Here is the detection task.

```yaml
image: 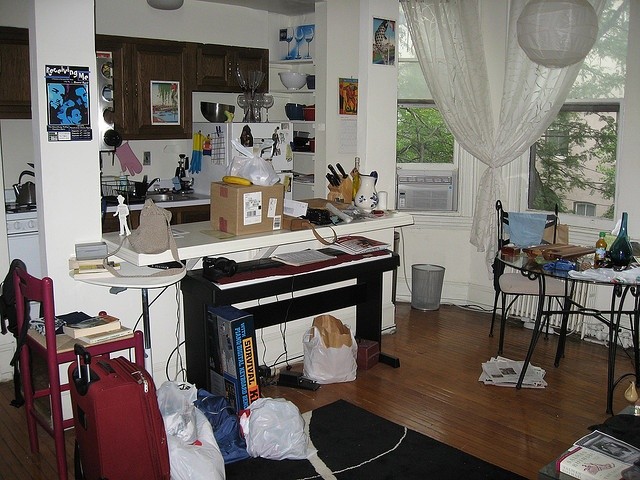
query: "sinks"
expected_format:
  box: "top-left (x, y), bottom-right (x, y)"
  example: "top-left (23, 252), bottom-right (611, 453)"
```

top-left (147, 194), bottom-right (190, 203)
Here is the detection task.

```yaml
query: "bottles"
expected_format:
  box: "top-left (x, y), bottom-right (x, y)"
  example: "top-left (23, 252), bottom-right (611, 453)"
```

top-left (594, 231), bottom-right (607, 269)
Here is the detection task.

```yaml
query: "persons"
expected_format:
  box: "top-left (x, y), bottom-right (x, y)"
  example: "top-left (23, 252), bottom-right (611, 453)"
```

top-left (48, 82), bottom-right (88, 124)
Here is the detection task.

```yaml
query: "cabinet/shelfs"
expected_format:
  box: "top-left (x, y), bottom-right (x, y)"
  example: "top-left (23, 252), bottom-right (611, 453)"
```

top-left (95, 33), bottom-right (187, 139)
top-left (0, 25), bottom-right (31, 119)
top-left (190, 41), bottom-right (269, 92)
top-left (268, 12), bottom-right (315, 202)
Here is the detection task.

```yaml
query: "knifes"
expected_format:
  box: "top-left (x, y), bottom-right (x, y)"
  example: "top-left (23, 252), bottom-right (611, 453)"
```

top-left (324, 162), bottom-right (353, 203)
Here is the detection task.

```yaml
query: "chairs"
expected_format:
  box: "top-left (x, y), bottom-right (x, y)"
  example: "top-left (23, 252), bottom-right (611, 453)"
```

top-left (12, 263), bottom-right (146, 480)
top-left (488, 200), bottom-right (574, 368)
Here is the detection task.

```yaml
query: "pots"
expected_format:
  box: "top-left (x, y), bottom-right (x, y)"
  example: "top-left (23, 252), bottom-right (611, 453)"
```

top-left (302, 104), bottom-right (315, 121)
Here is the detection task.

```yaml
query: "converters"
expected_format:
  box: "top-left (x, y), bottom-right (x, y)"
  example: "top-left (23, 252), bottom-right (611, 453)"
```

top-left (278, 370), bottom-right (303, 389)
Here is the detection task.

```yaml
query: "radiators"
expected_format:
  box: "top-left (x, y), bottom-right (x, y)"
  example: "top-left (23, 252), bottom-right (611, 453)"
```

top-left (503, 242), bottom-right (596, 339)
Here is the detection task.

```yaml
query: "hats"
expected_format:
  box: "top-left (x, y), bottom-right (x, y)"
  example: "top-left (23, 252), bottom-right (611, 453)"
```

top-left (300, 209), bottom-right (332, 224)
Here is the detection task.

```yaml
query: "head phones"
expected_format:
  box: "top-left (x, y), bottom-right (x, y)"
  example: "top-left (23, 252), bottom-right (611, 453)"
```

top-left (202, 256), bottom-right (238, 279)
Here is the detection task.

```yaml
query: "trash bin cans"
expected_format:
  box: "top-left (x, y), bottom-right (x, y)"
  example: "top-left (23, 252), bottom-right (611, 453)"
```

top-left (410, 263), bottom-right (446, 312)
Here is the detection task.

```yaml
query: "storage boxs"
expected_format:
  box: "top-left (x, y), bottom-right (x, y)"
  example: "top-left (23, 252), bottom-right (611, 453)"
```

top-left (210, 181), bottom-right (285, 236)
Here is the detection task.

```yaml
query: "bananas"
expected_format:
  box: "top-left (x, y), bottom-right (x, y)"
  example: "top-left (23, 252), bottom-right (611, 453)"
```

top-left (222, 176), bottom-right (253, 186)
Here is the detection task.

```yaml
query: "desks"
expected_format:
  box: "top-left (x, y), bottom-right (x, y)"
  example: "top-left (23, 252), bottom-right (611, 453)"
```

top-left (68, 260), bottom-right (188, 380)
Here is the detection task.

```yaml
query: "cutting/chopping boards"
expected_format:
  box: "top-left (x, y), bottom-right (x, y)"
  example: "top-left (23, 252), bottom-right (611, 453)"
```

top-left (145, 194), bottom-right (177, 202)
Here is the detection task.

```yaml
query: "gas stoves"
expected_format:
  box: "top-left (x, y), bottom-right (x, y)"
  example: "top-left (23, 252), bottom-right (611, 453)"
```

top-left (3, 188), bottom-right (42, 235)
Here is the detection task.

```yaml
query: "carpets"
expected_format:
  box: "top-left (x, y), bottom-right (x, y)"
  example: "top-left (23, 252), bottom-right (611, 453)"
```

top-left (225, 399), bottom-right (529, 480)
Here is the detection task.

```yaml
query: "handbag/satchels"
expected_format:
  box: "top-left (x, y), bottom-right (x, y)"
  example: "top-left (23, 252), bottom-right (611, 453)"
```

top-left (195, 389), bottom-right (250, 465)
top-left (309, 315), bottom-right (353, 348)
top-left (523, 243), bottom-right (596, 262)
top-left (126, 198), bottom-right (172, 254)
top-left (29, 316), bottom-right (67, 337)
top-left (281, 212), bottom-right (316, 231)
top-left (542, 214), bottom-right (568, 245)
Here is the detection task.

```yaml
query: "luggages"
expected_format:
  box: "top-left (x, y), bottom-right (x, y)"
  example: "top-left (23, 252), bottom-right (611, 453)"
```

top-left (68, 343), bottom-right (171, 480)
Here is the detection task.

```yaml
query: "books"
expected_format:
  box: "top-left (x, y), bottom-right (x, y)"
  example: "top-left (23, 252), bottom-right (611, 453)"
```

top-left (330, 231), bottom-right (391, 255)
top-left (72, 270), bottom-right (114, 279)
top-left (271, 248), bottom-right (338, 267)
top-left (574, 428), bottom-right (640, 466)
top-left (555, 445), bottom-right (633, 479)
top-left (78, 327), bottom-right (132, 345)
top-left (78, 262), bottom-right (121, 272)
top-left (62, 312), bottom-right (121, 339)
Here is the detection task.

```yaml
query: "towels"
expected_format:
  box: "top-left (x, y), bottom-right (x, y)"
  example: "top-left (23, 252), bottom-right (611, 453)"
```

top-left (503, 211), bottom-right (547, 248)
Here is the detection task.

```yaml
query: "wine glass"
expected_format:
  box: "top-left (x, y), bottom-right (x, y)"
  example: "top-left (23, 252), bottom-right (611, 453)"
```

top-left (253, 95), bottom-right (262, 123)
top-left (237, 95), bottom-right (248, 122)
top-left (236, 71), bottom-right (265, 122)
top-left (304, 28), bottom-right (313, 59)
top-left (263, 95), bottom-right (274, 122)
top-left (294, 27), bottom-right (303, 59)
top-left (286, 27), bottom-right (293, 60)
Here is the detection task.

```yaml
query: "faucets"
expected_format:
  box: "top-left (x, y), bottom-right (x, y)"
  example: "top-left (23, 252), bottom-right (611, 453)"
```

top-left (146, 178), bottom-right (160, 190)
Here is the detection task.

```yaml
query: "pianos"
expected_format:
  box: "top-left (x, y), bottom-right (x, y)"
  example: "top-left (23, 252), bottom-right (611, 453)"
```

top-left (181, 248), bottom-right (400, 391)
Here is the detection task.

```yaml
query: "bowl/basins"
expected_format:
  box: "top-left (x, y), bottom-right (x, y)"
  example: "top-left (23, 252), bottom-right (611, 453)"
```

top-left (284, 103), bottom-right (306, 120)
top-left (158, 188), bottom-right (170, 194)
top-left (200, 101), bottom-right (235, 123)
top-left (277, 72), bottom-right (307, 90)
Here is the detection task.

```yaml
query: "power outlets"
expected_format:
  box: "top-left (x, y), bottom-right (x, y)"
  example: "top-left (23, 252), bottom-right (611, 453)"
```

top-left (143, 151), bottom-right (150, 165)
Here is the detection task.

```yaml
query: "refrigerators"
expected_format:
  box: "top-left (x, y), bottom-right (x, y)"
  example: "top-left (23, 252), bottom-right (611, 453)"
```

top-left (192, 122), bottom-right (293, 199)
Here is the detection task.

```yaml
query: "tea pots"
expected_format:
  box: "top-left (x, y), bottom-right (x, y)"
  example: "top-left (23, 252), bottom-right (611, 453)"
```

top-left (13, 170), bottom-right (36, 205)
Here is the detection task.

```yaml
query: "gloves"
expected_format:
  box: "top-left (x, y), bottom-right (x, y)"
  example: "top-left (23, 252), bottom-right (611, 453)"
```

top-left (188, 133), bottom-right (206, 174)
top-left (115, 141), bottom-right (142, 176)
